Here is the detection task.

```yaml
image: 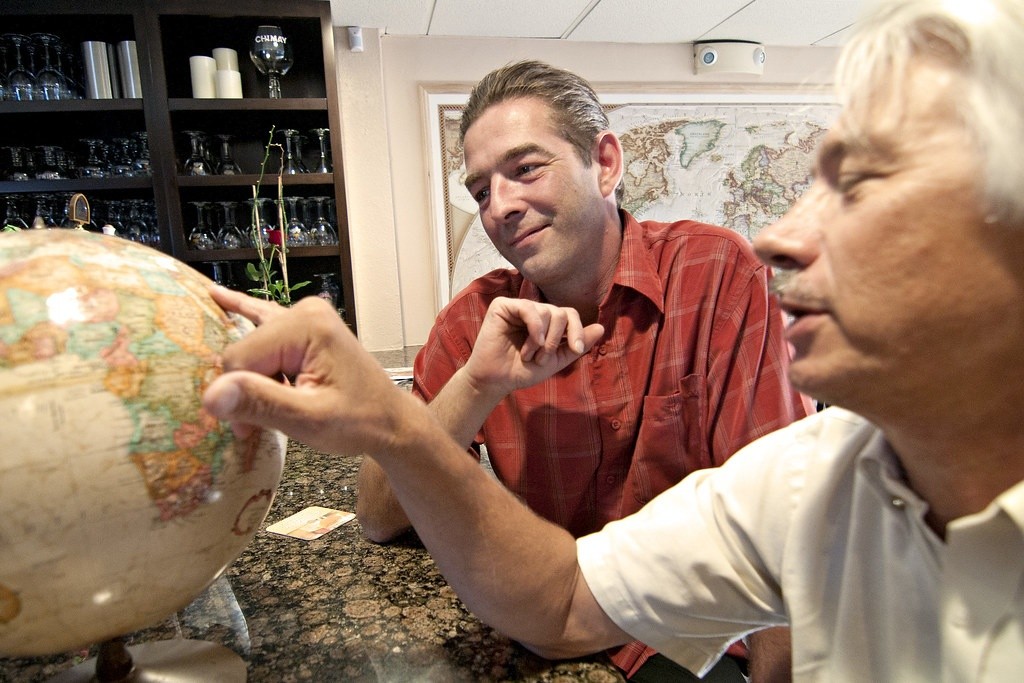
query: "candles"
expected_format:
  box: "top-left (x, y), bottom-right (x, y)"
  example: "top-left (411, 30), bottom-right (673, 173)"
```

top-left (212, 49), bottom-right (240, 72)
top-left (189, 56), bottom-right (217, 99)
top-left (215, 69), bottom-right (244, 99)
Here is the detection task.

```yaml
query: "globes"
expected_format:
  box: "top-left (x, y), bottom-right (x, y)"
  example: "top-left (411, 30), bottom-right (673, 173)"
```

top-left (0, 227), bottom-right (292, 683)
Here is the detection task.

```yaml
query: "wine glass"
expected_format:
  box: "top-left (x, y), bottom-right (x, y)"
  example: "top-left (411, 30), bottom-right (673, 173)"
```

top-left (0, 132), bottom-right (151, 179)
top-left (203, 262), bottom-right (355, 332)
top-left (182, 128), bottom-right (242, 177)
top-left (2, 194), bottom-right (157, 246)
top-left (190, 196), bottom-right (337, 247)
top-left (0, 33), bottom-right (85, 103)
top-left (276, 126), bottom-right (332, 174)
top-left (248, 24), bottom-right (291, 98)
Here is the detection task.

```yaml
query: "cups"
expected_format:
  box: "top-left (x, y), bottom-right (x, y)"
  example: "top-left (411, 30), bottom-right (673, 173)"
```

top-left (189, 47), bottom-right (244, 99)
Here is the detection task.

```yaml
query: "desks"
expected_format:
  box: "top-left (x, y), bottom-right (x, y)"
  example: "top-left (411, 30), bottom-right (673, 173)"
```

top-left (0, 438), bottom-right (633, 683)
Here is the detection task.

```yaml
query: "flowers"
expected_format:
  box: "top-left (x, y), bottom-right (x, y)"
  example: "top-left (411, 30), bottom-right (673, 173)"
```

top-left (244, 126), bottom-right (312, 306)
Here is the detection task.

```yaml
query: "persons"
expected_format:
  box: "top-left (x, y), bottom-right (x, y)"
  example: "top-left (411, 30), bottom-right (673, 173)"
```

top-left (204, 0), bottom-right (1024, 683)
top-left (357, 60), bottom-right (818, 683)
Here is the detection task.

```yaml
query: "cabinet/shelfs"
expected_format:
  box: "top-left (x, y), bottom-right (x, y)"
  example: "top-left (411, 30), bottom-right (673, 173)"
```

top-left (0, 0), bottom-right (358, 339)
